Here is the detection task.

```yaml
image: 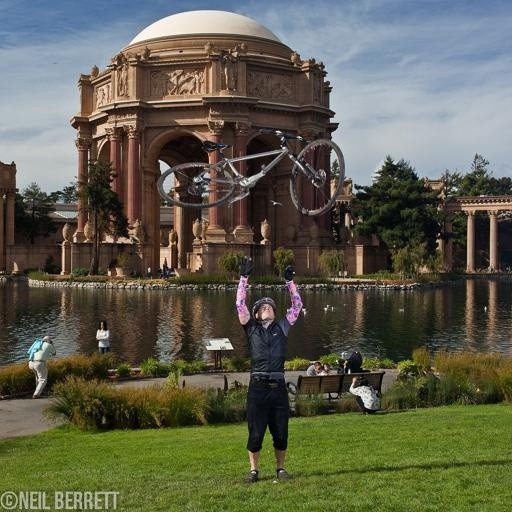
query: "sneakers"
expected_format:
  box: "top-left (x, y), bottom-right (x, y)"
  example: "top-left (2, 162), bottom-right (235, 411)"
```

top-left (249, 470), bottom-right (257, 482)
top-left (277, 468), bottom-right (290, 481)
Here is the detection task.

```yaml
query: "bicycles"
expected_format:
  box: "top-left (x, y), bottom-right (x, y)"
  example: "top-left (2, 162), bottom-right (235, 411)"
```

top-left (155, 124), bottom-right (346, 218)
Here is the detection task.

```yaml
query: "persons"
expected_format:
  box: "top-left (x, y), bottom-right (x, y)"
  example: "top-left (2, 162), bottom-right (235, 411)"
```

top-left (96, 320), bottom-right (111, 353)
top-left (235, 255), bottom-right (303, 482)
top-left (28, 336), bottom-right (56, 398)
top-left (349, 376), bottom-right (381, 415)
top-left (147, 265), bottom-right (170, 279)
top-left (307, 353), bottom-right (371, 378)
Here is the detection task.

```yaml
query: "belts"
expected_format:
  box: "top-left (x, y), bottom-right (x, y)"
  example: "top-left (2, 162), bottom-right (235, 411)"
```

top-left (254, 382), bottom-right (278, 388)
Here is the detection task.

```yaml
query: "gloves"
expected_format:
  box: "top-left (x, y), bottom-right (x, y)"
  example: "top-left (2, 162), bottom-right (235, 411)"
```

top-left (238, 255), bottom-right (255, 276)
top-left (284, 265), bottom-right (296, 280)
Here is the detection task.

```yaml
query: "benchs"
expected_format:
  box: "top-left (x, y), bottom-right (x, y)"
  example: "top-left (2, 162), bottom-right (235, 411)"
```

top-left (338, 370), bottom-right (387, 400)
top-left (285, 372), bottom-right (345, 406)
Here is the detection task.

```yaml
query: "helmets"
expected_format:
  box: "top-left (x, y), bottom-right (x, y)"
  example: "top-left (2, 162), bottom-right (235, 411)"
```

top-left (252, 297), bottom-right (277, 320)
